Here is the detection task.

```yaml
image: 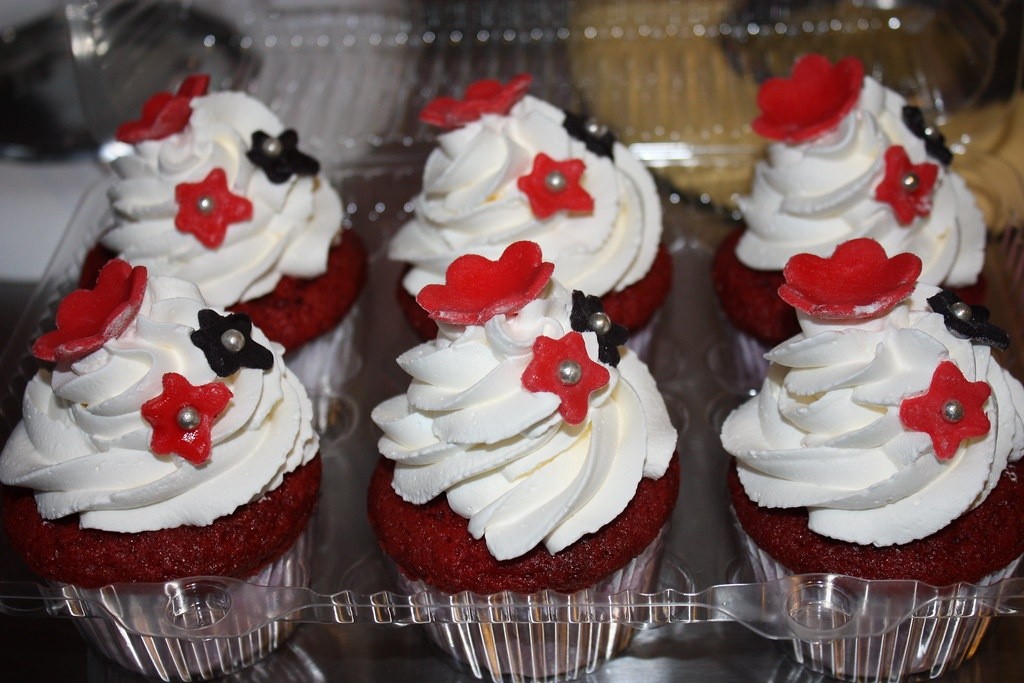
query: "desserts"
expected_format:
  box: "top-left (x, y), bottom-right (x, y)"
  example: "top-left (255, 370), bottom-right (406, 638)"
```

top-left (0, 53), bottom-right (1024, 677)
